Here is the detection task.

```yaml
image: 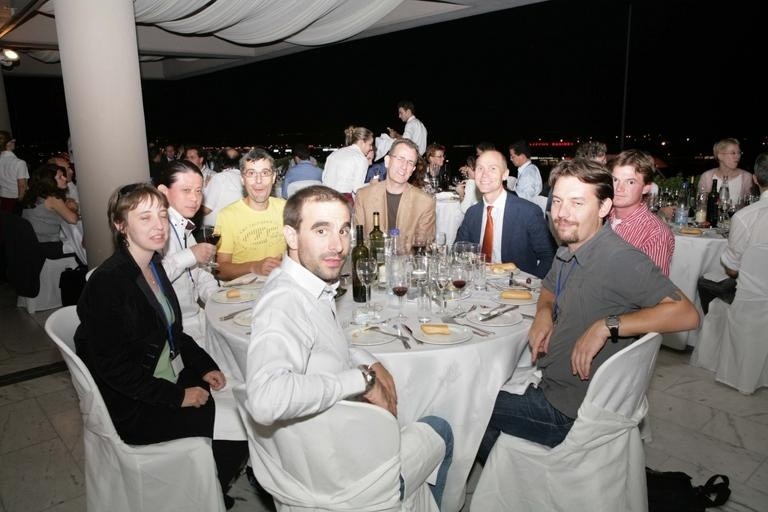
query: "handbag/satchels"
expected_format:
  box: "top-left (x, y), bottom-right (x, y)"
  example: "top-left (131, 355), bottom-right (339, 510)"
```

top-left (643, 464), bottom-right (732, 512)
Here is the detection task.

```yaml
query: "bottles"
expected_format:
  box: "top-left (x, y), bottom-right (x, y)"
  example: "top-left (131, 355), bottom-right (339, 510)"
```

top-left (424, 161), bottom-right (469, 200)
top-left (644, 175), bottom-right (755, 234)
top-left (351, 211), bottom-right (387, 303)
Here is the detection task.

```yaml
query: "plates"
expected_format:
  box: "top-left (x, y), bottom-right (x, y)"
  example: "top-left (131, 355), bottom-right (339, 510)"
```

top-left (495, 278), bottom-right (540, 290)
top-left (233, 308), bottom-right (253, 326)
top-left (493, 290), bottom-right (538, 306)
top-left (413, 323), bottom-right (473, 344)
top-left (213, 289), bottom-right (257, 303)
top-left (490, 263), bottom-right (520, 278)
top-left (345, 324), bottom-right (399, 345)
top-left (466, 308), bottom-right (522, 325)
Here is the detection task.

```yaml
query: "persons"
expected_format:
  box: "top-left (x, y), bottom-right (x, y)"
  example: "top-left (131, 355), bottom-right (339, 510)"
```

top-left (388, 100), bottom-right (429, 156)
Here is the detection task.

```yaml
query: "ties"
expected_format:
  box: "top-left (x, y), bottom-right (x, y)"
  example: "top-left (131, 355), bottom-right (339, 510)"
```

top-left (478, 205), bottom-right (498, 265)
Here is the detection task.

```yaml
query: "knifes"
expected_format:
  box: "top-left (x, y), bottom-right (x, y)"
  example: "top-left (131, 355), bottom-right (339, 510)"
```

top-left (401, 323), bottom-right (424, 345)
top-left (480, 305), bottom-right (519, 322)
top-left (509, 272), bottom-right (514, 285)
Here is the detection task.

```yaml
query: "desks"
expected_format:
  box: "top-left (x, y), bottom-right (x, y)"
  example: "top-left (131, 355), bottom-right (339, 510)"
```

top-left (60, 216), bottom-right (88, 265)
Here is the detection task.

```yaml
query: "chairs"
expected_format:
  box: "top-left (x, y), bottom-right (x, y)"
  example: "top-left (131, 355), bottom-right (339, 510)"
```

top-left (44, 304), bottom-right (227, 512)
top-left (286, 178), bottom-right (321, 201)
top-left (84, 261), bottom-right (103, 282)
top-left (688, 214), bottom-right (768, 394)
top-left (15, 216), bottom-right (77, 314)
top-left (230, 382), bottom-right (442, 511)
top-left (533, 195), bottom-right (549, 218)
top-left (469, 328), bottom-right (670, 511)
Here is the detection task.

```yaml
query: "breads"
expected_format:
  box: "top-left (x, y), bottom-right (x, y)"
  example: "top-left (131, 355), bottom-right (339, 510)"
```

top-left (680, 228), bottom-right (703, 234)
top-left (421, 324), bottom-right (450, 335)
top-left (227, 288), bottom-right (239, 298)
top-left (491, 262), bottom-right (515, 270)
top-left (502, 291), bottom-right (532, 299)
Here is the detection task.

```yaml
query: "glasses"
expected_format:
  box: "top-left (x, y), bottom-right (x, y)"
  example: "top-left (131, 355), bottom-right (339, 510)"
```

top-left (386, 152), bottom-right (416, 169)
top-left (241, 169), bottom-right (275, 179)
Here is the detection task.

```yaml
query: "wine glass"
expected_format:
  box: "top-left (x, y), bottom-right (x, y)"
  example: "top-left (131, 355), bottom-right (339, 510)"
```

top-left (203, 226), bottom-right (222, 268)
top-left (356, 228), bottom-right (489, 322)
